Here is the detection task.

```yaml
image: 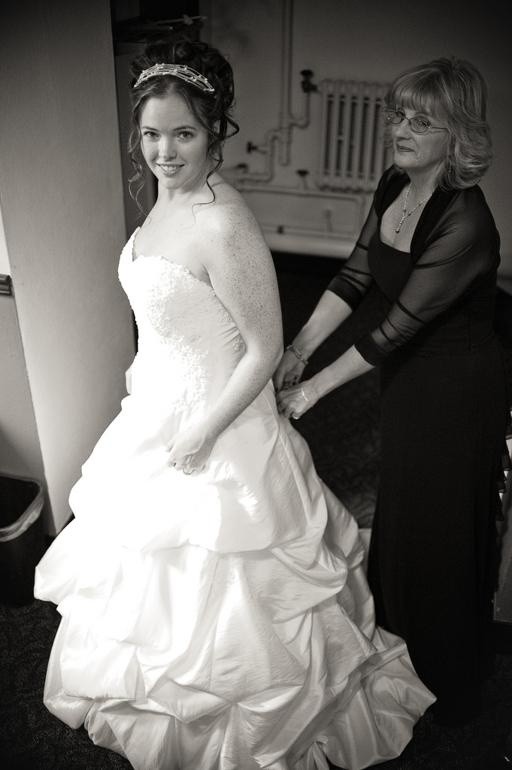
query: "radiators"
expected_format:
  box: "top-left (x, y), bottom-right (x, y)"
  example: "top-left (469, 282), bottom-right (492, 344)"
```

top-left (315, 79), bottom-right (392, 191)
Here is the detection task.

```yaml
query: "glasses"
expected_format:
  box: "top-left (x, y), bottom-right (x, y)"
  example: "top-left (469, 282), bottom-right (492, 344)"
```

top-left (386, 107), bottom-right (451, 136)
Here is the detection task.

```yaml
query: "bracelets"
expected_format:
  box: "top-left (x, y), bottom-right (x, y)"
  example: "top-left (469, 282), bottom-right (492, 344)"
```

top-left (286, 345), bottom-right (308, 365)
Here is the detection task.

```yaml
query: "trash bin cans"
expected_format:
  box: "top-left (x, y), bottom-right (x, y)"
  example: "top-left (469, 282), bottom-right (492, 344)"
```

top-left (0, 473), bottom-right (47, 608)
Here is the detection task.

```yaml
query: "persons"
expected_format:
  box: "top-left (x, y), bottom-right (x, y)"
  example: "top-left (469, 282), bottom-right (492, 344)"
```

top-left (273, 55), bottom-right (512, 729)
top-left (34, 20), bottom-right (438, 769)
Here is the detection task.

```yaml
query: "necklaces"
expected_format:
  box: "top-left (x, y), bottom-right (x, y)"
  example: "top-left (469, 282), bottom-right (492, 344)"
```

top-left (396, 181), bottom-right (432, 233)
